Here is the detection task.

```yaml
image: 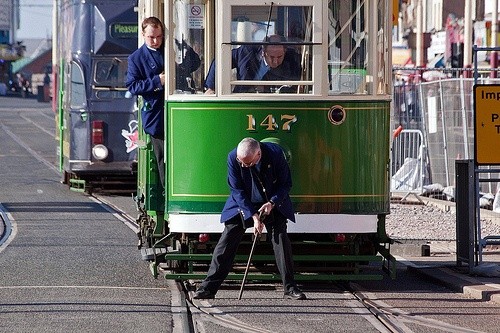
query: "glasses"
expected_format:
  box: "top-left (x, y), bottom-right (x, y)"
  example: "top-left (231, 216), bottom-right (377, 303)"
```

top-left (235, 155), bottom-right (257, 168)
top-left (148, 34), bottom-right (162, 39)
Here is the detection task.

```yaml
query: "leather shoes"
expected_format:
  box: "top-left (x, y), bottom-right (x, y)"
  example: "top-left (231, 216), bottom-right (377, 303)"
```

top-left (283, 288), bottom-right (306, 300)
top-left (193, 287), bottom-right (215, 299)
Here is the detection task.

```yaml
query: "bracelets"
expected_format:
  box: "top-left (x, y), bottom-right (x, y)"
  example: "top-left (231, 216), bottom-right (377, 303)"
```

top-left (269, 201), bottom-right (275, 206)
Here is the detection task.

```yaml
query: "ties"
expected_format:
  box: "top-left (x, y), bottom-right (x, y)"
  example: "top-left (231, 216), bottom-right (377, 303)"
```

top-left (250, 166), bottom-right (269, 201)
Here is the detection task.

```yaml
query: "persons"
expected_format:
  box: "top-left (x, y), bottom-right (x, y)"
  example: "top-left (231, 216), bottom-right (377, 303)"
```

top-left (125, 17), bottom-right (201, 189)
top-left (206, 34), bottom-right (302, 94)
top-left (193, 137), bottom-right (306, 300)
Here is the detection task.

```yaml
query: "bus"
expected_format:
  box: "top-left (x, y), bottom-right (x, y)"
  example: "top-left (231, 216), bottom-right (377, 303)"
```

top-left (52, 0), bottom-right (144, 190)
top-left (133, 0), bottom-right (393, 287)
